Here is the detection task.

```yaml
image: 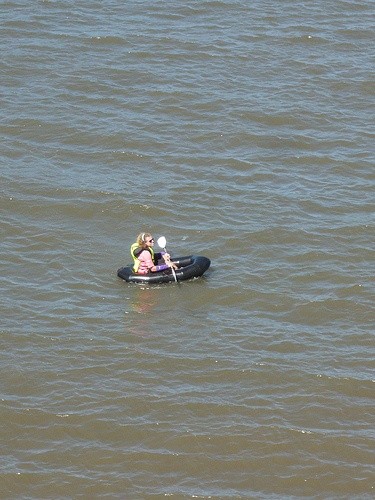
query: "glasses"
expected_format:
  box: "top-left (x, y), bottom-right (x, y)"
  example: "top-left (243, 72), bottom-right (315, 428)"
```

top-left (149, 238), bottom-right (154, 242)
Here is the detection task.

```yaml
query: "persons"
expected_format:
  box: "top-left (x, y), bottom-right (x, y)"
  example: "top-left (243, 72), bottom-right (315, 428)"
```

top-left (130, 232), bottom-right (178, 275)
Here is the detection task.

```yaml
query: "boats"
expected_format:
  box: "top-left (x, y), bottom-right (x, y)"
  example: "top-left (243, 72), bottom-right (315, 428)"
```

top-left (116, 255), bottom-right (211, 284)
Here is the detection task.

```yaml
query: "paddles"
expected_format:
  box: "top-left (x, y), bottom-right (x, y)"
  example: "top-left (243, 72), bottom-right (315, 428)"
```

top-left (157, 236), bottom-right (177, 283)
